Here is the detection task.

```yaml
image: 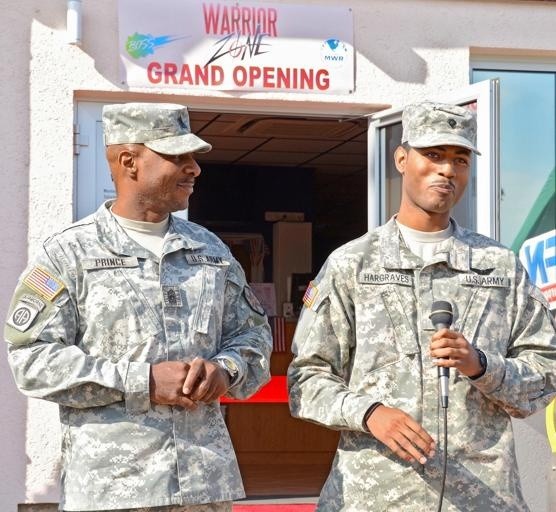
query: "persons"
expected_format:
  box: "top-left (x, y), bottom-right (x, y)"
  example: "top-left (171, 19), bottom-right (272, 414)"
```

top-left (285, 101), bottom-right (555, 510)
top-left (0, 101), bottom-right (276, 511)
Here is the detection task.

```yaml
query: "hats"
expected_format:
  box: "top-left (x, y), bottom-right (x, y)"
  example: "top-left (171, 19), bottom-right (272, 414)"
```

top-left (101, 103), bottom-right (213, 157)
top-left (402, 101), bottom-right (483, 157)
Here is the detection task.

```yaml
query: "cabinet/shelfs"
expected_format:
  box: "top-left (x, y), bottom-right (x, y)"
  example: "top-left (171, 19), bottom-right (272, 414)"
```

top-left (219, 349), bottom-right (342, 500)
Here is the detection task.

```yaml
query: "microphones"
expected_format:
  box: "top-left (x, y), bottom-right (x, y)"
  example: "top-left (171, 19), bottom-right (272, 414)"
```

top-left (429, 299), bottom-right (453, 412)
